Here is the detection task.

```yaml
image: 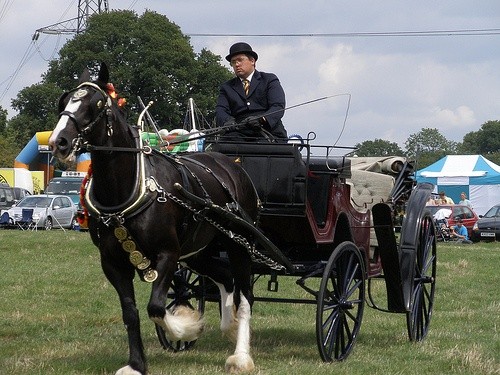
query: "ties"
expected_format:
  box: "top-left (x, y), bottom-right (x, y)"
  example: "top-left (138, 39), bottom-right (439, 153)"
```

top-left (244, 80), bottom-right (250, 96)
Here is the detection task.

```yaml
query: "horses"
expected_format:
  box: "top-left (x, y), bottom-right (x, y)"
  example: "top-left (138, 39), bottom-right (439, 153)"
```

top-left (45, 58), bottom-right (271, 375)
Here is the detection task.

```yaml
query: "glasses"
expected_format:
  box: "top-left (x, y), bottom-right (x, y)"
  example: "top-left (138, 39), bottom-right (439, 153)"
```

top-left (440, 194), bottom-right (444, 196)
top-left (231, 58), bottom-right (251, 65)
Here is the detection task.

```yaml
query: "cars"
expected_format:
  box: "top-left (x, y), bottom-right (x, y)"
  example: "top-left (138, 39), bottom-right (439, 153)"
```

top-left (425, 205), bottom-right (480, 243)
top-left (471, 204), bottom-right (500, 242)
top-left (5, 194), bottom-right (78, 231)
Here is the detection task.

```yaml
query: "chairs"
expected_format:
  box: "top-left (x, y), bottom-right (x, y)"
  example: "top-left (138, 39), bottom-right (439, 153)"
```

top-left (0, 208), bottom-right (38, 231)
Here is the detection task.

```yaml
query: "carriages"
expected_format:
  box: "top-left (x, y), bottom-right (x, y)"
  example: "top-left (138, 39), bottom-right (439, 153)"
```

top-left (47, 61), bottom-right (436, 375)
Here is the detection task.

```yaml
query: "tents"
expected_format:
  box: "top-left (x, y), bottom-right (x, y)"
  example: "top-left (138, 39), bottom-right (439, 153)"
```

top-left (408, 154), bottom-right (500, 219)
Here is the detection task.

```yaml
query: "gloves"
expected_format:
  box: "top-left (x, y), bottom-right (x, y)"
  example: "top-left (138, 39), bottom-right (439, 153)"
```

top-left (224, 120), bottom-right (239, 130)
top-left (242, 116), bottom-right (260, 127)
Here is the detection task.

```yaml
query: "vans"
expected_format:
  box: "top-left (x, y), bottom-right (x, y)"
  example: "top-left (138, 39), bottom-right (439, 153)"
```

top-left (0, 186), bottom-right (33, 217)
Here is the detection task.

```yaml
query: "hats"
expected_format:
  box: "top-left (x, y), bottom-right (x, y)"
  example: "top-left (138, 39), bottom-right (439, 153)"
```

top-left (225, 43), bottom-right (258, 62)
top-left (454, 217), bottom-right (460, 221)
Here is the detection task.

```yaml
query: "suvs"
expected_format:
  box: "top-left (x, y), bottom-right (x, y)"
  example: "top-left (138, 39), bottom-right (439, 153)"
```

top-left (42, 171), bottom-right (86, 215)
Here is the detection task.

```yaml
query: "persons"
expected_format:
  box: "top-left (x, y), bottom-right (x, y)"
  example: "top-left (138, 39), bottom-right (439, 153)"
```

top-left (440, 219), bottom-right (468, 241)
top-left (426, 192), bottom-right (473, 218)
top-left (216, 42), bottom-right (289, 144)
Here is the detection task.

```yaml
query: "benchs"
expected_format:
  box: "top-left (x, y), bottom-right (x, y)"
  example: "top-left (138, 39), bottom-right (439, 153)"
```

top-left (303, 158), bottom-right (351, 225)
top-left (346, 155), bottom-right (409, 249)
